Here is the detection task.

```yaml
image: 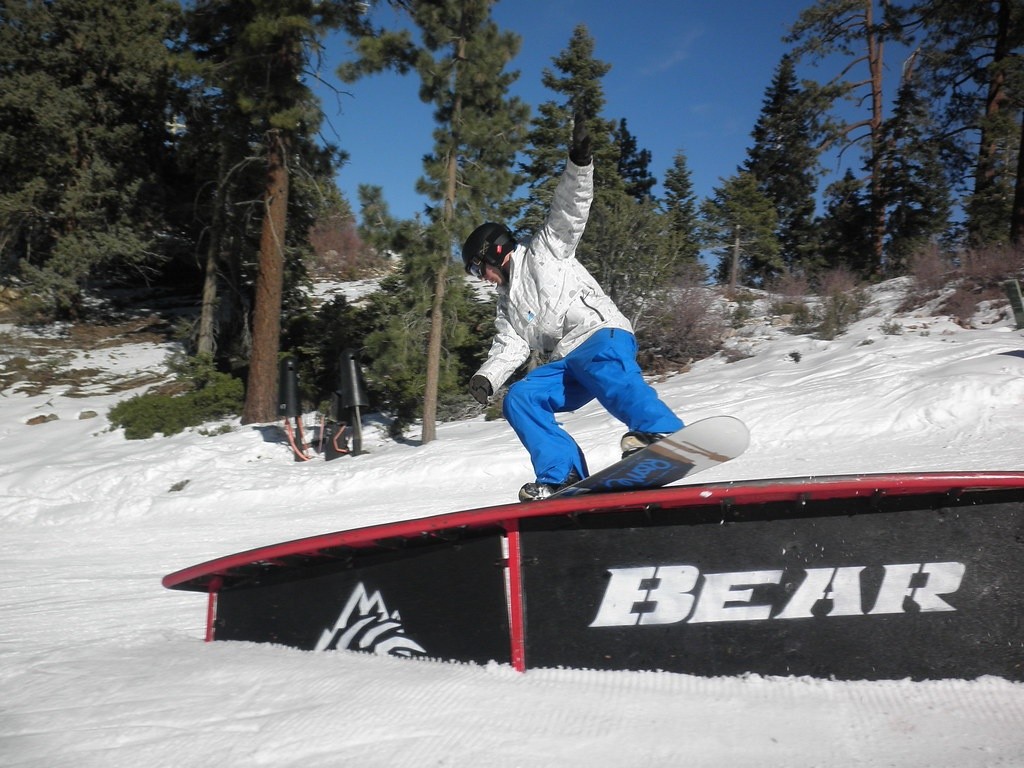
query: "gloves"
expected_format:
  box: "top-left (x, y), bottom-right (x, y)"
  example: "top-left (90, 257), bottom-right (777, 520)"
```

top-left (570, 112), bottom-right (592, 165)
top-left (468, 375), bottom-right (493, 405)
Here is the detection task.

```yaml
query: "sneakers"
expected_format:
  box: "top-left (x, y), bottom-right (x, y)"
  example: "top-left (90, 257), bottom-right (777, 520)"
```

top-left (518, 467), bottom-right (581, 502)
top-left (621, 430), bottom-right (672, 459)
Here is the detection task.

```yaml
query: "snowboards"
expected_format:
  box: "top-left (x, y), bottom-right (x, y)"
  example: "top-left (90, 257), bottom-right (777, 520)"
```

top-left (546, 415), bottom-right (751, 499)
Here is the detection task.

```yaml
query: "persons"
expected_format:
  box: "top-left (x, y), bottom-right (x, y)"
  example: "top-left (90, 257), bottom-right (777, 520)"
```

top-left (462, 111), bottom-right (684, 502)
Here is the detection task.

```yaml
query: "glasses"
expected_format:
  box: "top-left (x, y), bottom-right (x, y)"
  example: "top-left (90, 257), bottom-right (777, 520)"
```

top-left (465, 255), bottom-right (486, 280)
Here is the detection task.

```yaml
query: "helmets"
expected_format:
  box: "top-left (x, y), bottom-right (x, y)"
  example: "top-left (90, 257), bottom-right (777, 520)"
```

top-left (462, 222), bottom-right (518, 266)
